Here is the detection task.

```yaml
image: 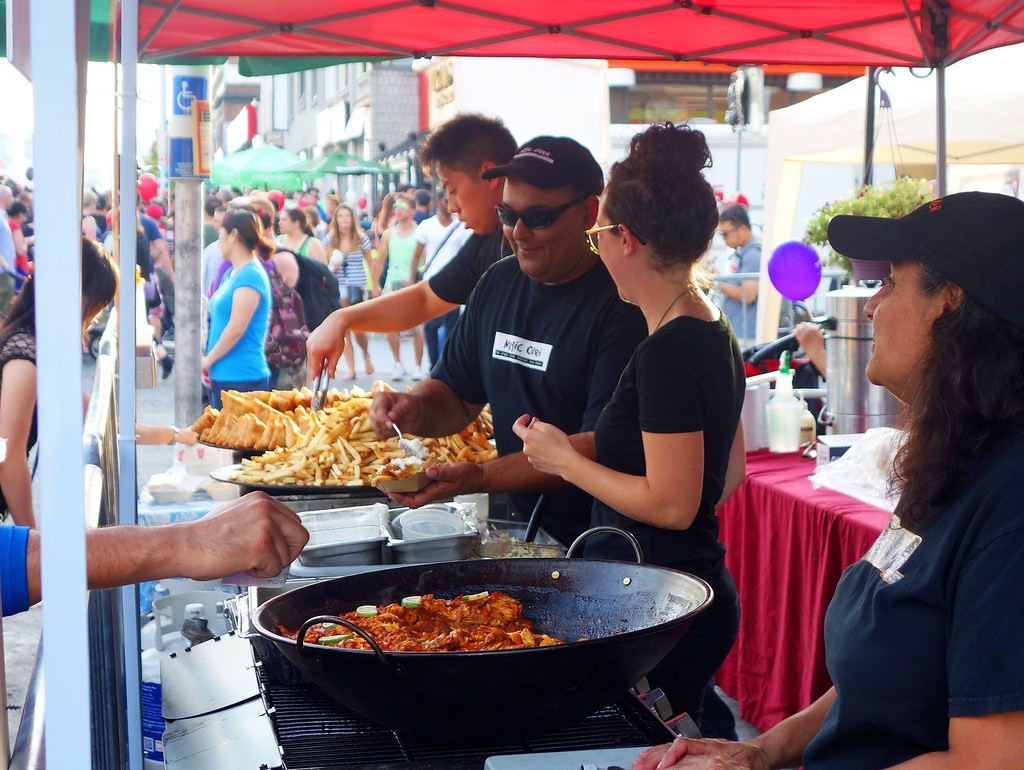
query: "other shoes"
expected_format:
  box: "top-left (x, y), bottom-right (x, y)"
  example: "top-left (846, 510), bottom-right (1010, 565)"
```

top-left (341, 370), bottom-right (356, 381)
top-left (391, 361), bottom-right (405, 381)
top-left (363, 352), bottom-right (373, 374)
top-left (412, 363), bottom-right (422, 380)
top-left (161, 353), bottom-right (174, 379)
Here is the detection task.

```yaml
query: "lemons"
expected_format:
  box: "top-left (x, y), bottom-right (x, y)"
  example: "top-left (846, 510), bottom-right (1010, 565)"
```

top-left (461, 591), bottom-right (489, 601)
top-left (401, 596), bottom-right (422, 608)
top-left (319, 635), bottom-right (353, 645)
top-left (356, 605), bottom-right (377, 616)
top-left (323, 623), bottom-right (337, 628)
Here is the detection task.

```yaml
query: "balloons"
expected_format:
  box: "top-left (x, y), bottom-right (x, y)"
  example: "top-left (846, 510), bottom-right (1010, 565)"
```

top-left (768, 242), bottom-right (823, 303)
top-left (357, 197), bottom-right (366, 210)
top-left (268, 191), bottom-right (285, 211)
top-left (137, 174), bottom-right (160, 201)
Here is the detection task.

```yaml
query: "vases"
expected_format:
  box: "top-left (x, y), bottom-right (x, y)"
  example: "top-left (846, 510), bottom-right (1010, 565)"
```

top-left (849, 258), bottom-right (890, 288)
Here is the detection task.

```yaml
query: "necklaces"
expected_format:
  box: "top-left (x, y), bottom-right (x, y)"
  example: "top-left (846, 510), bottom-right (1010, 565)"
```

top-left (652, 284), bottom-right (697, 333)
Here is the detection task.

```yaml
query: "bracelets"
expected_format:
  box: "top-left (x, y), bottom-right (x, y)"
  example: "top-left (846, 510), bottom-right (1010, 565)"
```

top-left (168, 425), bottom-right (181, 446)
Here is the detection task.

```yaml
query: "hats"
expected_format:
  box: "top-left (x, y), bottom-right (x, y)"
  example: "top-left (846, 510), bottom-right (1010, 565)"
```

top-left (827, 190), bottom-right (1024, 336)
top-left (480, 136), bottom-right (603, 196)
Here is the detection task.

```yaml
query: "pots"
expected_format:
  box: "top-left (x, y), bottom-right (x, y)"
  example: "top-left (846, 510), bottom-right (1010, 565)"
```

top-left (250, 526), bottom-right (715, 724)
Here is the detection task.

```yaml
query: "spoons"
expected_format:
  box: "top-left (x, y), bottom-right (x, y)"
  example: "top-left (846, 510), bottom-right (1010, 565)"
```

top-left (392, 422), bottom-right (413, 454)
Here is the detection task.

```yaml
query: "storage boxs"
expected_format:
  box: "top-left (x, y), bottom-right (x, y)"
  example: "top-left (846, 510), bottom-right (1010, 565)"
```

top-left (294, 502), bottom-right (388, 568)
top-left (815, 433), bottom-right (862, 466)
top-left (377, 503), bottom-right (476, 562)
top-left (471, 518), bottom-right (568, 557)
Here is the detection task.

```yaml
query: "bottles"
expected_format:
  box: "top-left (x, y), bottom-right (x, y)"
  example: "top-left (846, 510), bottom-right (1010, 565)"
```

top-left (799, 393), bottom-right (817, 445)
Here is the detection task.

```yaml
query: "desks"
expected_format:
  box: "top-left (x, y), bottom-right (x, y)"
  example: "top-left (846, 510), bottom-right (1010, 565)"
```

top-left (715, 449), bottom-right (893, 732)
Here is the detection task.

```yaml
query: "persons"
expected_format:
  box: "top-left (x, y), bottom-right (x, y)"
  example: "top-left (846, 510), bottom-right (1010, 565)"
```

top-left (0, 166), bottom-right (475, 412)
top-left (792, 321), bottom-right (827, 436)
top-left (695, 205), bottom-right (761, 339)
top-left (511, 121), bottom-right (743, 743)
top-left (0, 491), bottom-right (310, 617)
top-left (631, 191), bottom-right (1024, 770)
top-left (370, 136), bottom-right (647, 556)
top-left (0, 236), bottom-right (197, 530)
top-left (305, 113), bottom-right (519, 380)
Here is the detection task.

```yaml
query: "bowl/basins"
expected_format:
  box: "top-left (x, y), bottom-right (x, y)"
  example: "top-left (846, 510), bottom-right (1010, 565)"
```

top-left (200, 479), bottom-right (238, 500)
top-left (399, 509), bottom-right (465, 541)
top-left (145, 471), bottom-right (198, 503)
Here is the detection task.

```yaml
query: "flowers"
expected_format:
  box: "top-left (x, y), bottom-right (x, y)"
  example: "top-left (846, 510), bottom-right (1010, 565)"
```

top-left (800, 176), bottom-right (935, 243)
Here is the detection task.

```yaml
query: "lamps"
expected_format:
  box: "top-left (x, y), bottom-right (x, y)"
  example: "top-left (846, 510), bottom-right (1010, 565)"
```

top-left (608, 68), bottom-right (637, 90)
top-left (785, 72), bottom-right (823, 93)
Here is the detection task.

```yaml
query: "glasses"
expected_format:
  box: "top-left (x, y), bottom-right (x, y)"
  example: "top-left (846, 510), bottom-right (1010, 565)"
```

top-left (719, 222), bottom-right (744, 238)
top-left (584, 223), bottom-right (647, 255)
top-left (494, 191), bottom-right (592, 229)
top-left (392, 202), bottom-right (409, 211)
top-left (309, 192), bottom-right (319, 201)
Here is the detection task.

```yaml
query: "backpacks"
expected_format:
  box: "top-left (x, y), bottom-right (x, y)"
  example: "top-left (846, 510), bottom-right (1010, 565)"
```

top-left (259, 259), bottom-right (309, 369)
top-left (296, 250), bottom-right (341, 332)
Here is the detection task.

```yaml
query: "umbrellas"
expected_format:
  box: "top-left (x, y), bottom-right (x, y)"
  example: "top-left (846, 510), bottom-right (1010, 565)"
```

top-left (206, 143), bottom-right (400, 192)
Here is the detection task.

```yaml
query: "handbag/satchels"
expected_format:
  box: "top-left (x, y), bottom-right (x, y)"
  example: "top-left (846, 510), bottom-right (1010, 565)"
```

top-left (346, 285), bottom-right (363, 305)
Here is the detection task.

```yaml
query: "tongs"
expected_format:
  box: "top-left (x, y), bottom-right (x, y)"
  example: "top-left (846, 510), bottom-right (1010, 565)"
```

top-left (310, 357), bottom-right (330, 411)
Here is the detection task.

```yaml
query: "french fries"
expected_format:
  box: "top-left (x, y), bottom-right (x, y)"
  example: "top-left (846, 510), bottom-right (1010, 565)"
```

top-left (235, 398), bottom-right (497, 486)
